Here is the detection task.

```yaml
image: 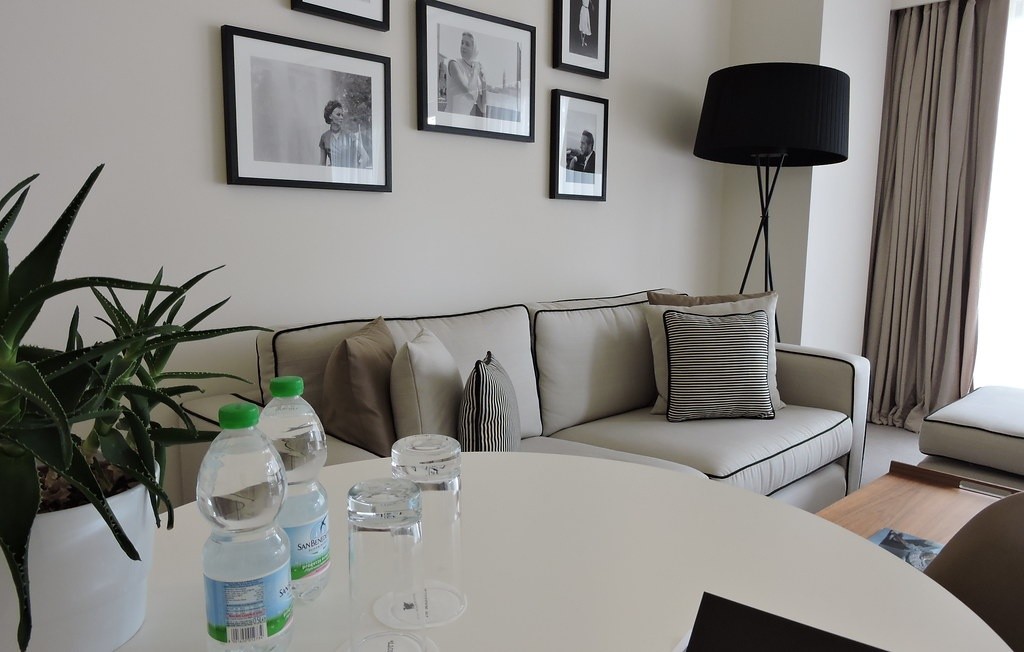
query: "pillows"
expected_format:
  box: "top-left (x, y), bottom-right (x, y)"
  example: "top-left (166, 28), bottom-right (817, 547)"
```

top-left (647, 290), bottom-right (785, 407)
top-left (457, 350), bottom-right (521, 452)
top-left (662, 314), bottom-right (776, 422)
top-left (322, 316), bottom-right (396, 457)
top-left (390, 328), bottom-right (464, 441)
top-left (646, 293), bottom-right (786, 415)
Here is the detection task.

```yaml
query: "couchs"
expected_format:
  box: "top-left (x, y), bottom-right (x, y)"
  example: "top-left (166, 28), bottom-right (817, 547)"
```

top-left (179, 287), bottom-right (872, 514)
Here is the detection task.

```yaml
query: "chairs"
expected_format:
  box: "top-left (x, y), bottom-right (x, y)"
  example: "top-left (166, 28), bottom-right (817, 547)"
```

top-left (921, 491), bottom-right (1024, 652)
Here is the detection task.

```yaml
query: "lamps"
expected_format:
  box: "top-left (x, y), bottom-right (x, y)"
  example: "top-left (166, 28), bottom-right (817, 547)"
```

top-left (694, 62), bottom-right (849, 343)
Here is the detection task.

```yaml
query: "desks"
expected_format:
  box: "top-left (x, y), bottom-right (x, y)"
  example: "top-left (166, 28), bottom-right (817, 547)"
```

top-left (115, 451), bottom-right (1014, 652)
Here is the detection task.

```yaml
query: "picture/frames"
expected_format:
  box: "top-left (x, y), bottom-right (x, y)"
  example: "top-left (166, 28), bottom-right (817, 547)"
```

top-left (220, 25), bottom-right (392, 192)
top-left (291, 0), bottom-right (390, 32)
top-left (548, 90), bottom-right (609, 201)
top-left (416, 0), bottom-right (537, 143)
top-left (552, 0), bottom-right (612, 79)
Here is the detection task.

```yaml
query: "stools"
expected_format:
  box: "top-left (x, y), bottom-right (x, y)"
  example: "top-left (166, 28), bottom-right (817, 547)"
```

top-left (916, 385), bottom-right (1024, 498)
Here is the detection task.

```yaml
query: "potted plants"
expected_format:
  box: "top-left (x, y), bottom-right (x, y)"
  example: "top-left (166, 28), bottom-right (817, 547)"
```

top-left (0, 162), bottom-right (274, 652)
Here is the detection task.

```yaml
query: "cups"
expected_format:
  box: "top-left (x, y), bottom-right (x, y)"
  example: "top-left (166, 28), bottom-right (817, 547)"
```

top-left (394, 439), bottom-right (463, 569)
top-left (347, 479), bottom-right (421, 605)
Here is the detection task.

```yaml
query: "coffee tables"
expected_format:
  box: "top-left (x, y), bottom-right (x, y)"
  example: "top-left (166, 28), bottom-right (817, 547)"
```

top-left (813, 460), bottom-right (1024, 570)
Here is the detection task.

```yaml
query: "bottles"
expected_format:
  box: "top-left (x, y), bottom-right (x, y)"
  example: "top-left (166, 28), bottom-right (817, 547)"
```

top-left (257, 377), bottom-right (331, 583)
top-left (197, 403), bottom-right (295, 652)
